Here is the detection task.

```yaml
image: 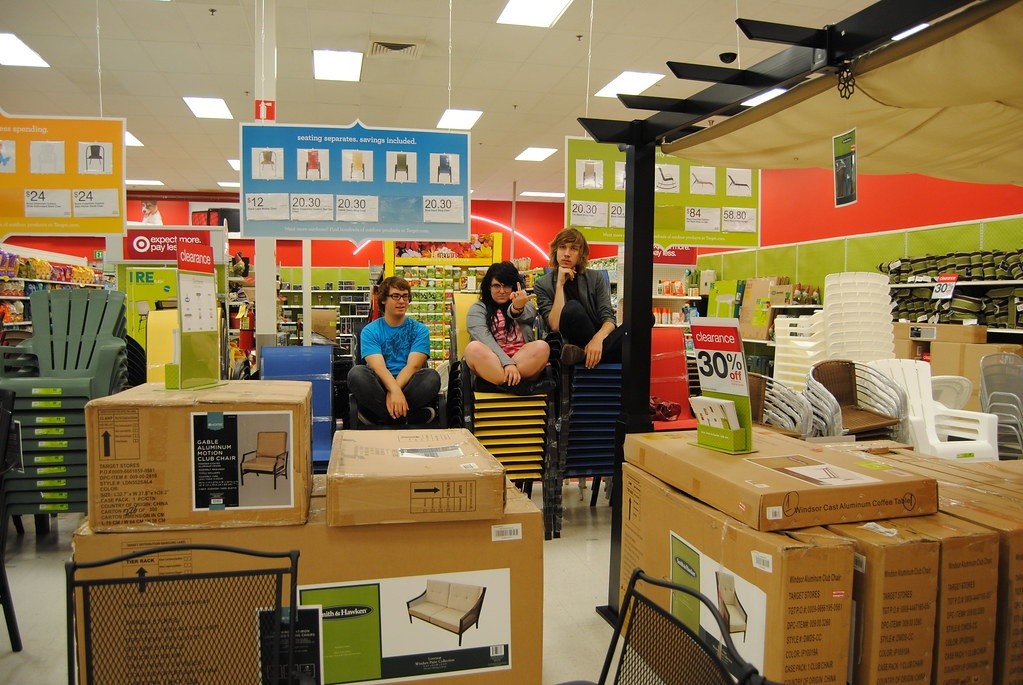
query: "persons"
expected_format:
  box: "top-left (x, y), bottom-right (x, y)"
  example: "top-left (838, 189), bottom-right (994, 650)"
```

top-left (463, 260), bottom-right (557, 396)
top-left (347, 277), bottom-right (441, 427)
top-left (534, 228), bottom-right (623, 369)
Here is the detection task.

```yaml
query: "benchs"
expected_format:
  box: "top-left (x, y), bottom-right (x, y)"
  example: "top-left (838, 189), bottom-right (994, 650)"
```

top-left (406, 579), bottom-right (487, 647)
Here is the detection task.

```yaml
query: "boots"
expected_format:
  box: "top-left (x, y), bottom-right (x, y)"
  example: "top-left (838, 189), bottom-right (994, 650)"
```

top-left (500, 379), bottom-right (556, 395)
top-left (475, 376), bottom-right (500, 392)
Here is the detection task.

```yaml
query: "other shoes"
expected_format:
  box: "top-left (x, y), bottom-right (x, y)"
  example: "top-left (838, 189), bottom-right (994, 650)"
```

top-left (406, 406), bottom-right (435, 425)
top-left (560, 344), bottom-right (586, 365)
top-left (357, 409), bottom-right (381, 425)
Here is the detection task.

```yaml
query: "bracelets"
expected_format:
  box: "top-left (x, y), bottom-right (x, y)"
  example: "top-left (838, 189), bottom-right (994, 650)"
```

top-left (513, 305), bottom-right (524, 312)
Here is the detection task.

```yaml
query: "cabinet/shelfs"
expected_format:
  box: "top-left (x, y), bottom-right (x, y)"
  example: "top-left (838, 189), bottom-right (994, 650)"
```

top-left (218, 278), bottom-right (371, 379)
top-left (0, 275), bottom-right (106, 348)
top-left (382, 233), bottom-right (702, 430)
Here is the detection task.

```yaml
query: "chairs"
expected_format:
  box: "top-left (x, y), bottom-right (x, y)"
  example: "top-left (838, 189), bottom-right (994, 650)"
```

top-left (551, 568), bottom-right (782, 685)
top-left (687, 271), bottom-right (1023, 460)
top-left (714, 571), bottom-right (748, 643)
top-left (0, 289), bottom-right (147, 652)
top-left (63, 543), bottom-right (317, 685)
top-left (350, 294), bottom-right (621, 541)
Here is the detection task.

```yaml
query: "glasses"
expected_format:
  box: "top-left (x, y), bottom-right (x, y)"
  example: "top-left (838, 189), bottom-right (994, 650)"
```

top-left (388, 293), bottom-right (410, 301)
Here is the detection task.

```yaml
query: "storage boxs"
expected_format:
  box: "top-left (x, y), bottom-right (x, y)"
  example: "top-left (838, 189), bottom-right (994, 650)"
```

top-left (618, 325), bottom-right (1023, 685)
top-left (71, 379), bottom-right (543, 685)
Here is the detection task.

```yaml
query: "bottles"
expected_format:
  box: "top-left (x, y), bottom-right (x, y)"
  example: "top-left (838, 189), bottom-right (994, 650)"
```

top-left (658, 280), bottom-right (662, 295)
